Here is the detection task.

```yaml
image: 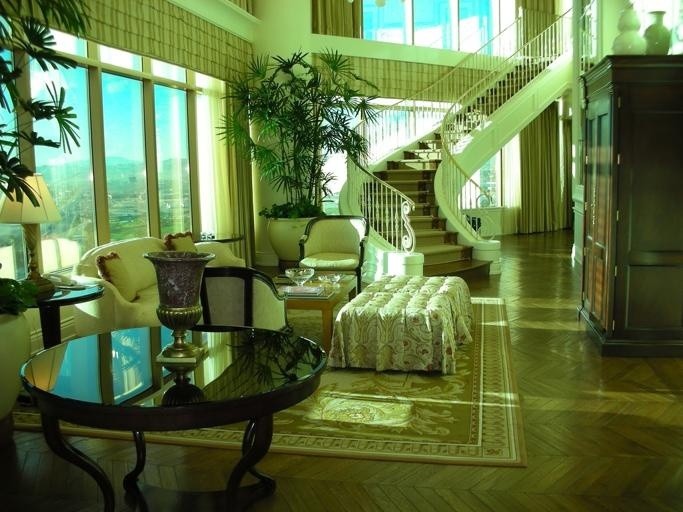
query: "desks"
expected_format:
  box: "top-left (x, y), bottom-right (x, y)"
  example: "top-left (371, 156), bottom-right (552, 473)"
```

top-left (20, 325), bottom-right (327, 512)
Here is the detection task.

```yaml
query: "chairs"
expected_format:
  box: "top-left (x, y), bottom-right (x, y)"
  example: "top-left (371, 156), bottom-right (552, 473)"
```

top-left (297, 215), bottom-right (370, 294)
top-left (200, 267), bottom-right (289, 332)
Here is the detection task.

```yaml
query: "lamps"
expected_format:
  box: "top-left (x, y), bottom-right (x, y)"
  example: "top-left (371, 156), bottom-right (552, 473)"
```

top-left (0, 173), bottom-right (62, 295)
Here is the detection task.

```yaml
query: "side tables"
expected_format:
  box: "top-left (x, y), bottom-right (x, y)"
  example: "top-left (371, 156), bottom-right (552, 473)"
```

top-left (193, 232), bottom-right (249, 268)
top-left (21, 282), bottom-right (105, 405)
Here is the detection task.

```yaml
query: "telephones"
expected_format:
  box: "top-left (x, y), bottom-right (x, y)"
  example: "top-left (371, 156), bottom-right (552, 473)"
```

top-left (42, 273), bottom-right (76, 286)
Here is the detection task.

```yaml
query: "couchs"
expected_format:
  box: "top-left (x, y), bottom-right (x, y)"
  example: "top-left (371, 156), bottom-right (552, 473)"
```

top-left (71, 236), bottom-right (246, 337)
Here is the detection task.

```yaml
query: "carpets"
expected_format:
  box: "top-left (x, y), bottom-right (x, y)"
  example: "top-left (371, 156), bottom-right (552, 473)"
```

top-left (12, 296), bottom-right (529, 469)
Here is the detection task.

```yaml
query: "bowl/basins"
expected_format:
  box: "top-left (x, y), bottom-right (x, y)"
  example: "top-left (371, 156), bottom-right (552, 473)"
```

top-left (318, 275), bottom-right (344, 284)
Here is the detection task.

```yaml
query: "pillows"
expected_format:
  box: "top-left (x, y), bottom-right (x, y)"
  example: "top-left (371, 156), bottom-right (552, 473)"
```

top-left (95, 250), bottom-right (138, 303)
top-left (164, 231), bottom-right (199, 254)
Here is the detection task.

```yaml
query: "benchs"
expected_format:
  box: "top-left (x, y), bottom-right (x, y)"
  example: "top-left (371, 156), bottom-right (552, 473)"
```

top-left (328, 273), bottom-right (475, 374)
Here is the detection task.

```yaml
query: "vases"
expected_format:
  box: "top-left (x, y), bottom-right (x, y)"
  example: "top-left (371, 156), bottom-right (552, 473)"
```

top-left (644, 10), bottom-right (671, 54)
top-left (158, 362), bottom-right (206, 406)
top-left (612, 8), bottom-right (647, 54)
top-left (141, 250), bottom-right (215, 358)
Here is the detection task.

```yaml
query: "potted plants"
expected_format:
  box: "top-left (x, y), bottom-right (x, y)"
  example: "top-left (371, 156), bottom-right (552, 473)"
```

top-left (214, 47), bottom-right (382, 271)
top-left (0, 0), bottom-right (93, 448)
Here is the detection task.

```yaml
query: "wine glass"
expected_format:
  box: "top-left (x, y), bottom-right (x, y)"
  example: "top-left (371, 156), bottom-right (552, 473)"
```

top-left (285, 268), bottom-right (315, 287)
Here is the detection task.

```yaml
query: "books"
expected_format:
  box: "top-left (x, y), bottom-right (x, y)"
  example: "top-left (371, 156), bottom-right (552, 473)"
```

top-left (286, 290), bottom-right (335, 300)
top-left (276, 285), bottom-right (324, 296)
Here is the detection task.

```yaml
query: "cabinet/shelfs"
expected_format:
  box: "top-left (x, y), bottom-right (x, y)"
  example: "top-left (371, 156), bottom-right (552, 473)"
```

top-left (575, 54), bottom-right (682, 357)
